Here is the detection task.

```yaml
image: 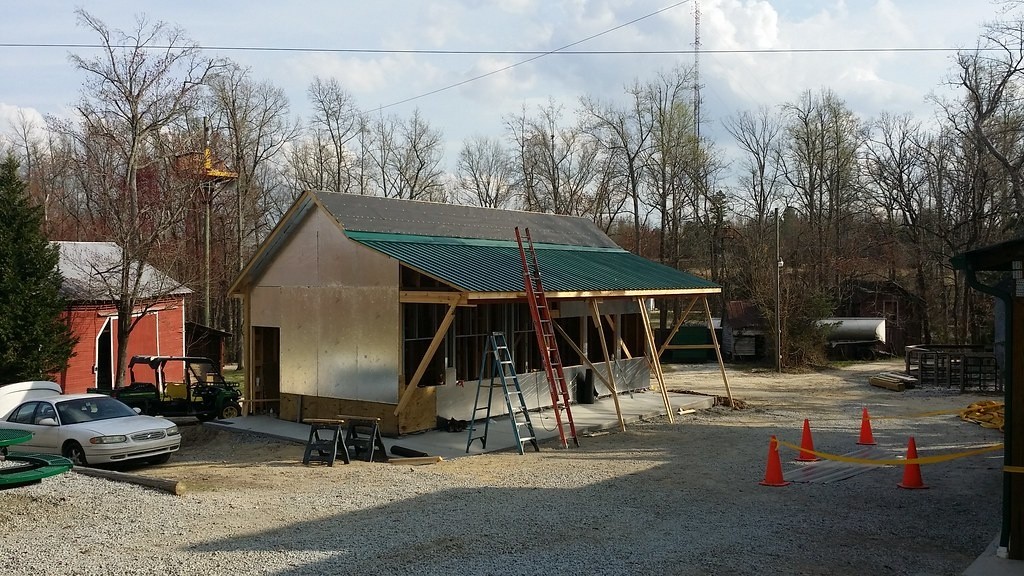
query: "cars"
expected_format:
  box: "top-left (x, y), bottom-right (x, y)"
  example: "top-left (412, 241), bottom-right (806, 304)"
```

top-left (0, 380), bottom-right (182, 468)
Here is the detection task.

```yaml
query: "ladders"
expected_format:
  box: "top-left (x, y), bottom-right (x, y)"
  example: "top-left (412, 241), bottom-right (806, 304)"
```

top-left (465, 331), bottom-right (541, 456)
top-left (512, 225), bottom-right (582, 450)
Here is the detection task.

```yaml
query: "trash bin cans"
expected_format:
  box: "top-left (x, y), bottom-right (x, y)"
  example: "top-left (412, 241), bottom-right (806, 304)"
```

top-left (576, 368), bottom-right (595, 404)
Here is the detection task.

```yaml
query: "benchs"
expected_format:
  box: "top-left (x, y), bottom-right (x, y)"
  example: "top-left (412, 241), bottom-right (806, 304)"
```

top-left (238, 399), bottom-right (280, 417)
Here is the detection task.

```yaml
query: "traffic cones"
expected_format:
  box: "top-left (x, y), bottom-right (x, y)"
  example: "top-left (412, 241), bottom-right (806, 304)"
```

top-left (758, 434), bottom-right (791, 487)
top-left (856, 407), bottom-right (878, 444)
top-left (795, 418), bottom-right (820, 461)
top-left (896, 436), bottom-right (931, 490)
